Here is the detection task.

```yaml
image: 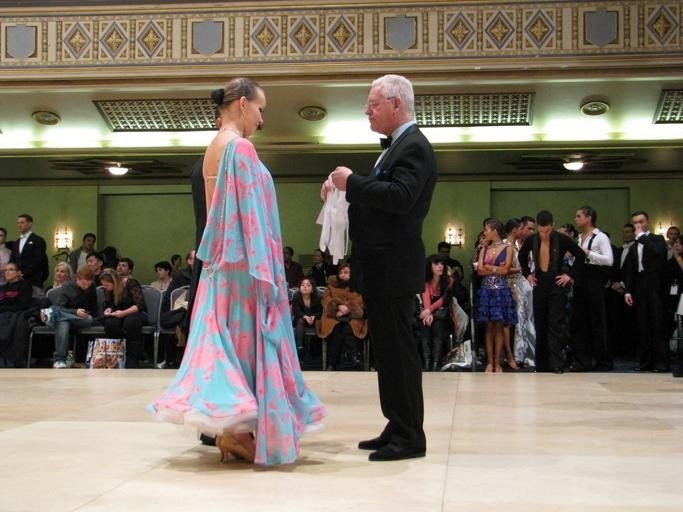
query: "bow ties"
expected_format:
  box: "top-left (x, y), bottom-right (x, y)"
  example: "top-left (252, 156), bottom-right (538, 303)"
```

top-left (380, 136), bottom-right (394, 150)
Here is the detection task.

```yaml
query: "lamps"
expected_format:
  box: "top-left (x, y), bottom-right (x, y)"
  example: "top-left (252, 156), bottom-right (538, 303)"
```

top-left (53, 224), bottom-right (74, 251)
top-left (99, 160), bottom-right (132, 176)
top-left (561, 153), bottom-right (586, 172)
top-left (442, 216), bottom-right (467, 252)
top-left (653, 221), bottom-right (674, 242)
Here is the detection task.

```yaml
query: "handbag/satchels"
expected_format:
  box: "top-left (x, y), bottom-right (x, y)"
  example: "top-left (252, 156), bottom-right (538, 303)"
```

top-left (86, 337), bottom-right (127, 369)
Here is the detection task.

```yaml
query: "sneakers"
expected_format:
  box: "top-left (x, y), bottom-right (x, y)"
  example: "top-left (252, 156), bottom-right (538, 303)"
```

top-left (53, 361), bottom-right (67, 368)
top-left (41, 308), bottom-right (52, 323)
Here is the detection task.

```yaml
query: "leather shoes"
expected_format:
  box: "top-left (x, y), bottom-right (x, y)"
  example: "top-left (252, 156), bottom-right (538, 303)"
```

top-left (353, 353), bottom-right (362, 364)
top-left (327, 364), bottom-right (334, 371)
top-left (652, 367), bottom-right (669, 372)
top-left (634, 366), bottom-right (649, 371)
top-left (370, 443), bottom-right (426, 461)
top-left (359, 437), bottom-right (391, 450)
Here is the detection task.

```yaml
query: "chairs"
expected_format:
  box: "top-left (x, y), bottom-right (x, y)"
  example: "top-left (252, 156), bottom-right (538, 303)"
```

top-left (0, 258), bottom-right (475, 368)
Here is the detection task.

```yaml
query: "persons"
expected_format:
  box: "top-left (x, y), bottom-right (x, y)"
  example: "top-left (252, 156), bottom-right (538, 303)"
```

top-left (282, 246), bottom-right (376, 372)
top-left (1, 214), bottom-right (197, 368)
top-left (320, 75), bottom-right (438, 460)
top-left (148, 77), bottom-right (328, 466)
top-left (419, 206), bottom-right (683, 376)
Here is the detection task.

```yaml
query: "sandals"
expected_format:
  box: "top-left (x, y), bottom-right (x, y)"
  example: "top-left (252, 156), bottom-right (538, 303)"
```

top-left (503, 357), bottom-right (520, 369)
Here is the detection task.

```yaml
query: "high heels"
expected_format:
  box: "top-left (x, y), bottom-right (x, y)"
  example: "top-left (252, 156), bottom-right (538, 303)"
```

top-left (215, 432), bottom-right (257, 464)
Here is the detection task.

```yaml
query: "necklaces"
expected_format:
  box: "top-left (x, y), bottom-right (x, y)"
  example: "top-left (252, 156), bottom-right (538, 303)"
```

top-left (216, 128), bottom-right (241, 136)
